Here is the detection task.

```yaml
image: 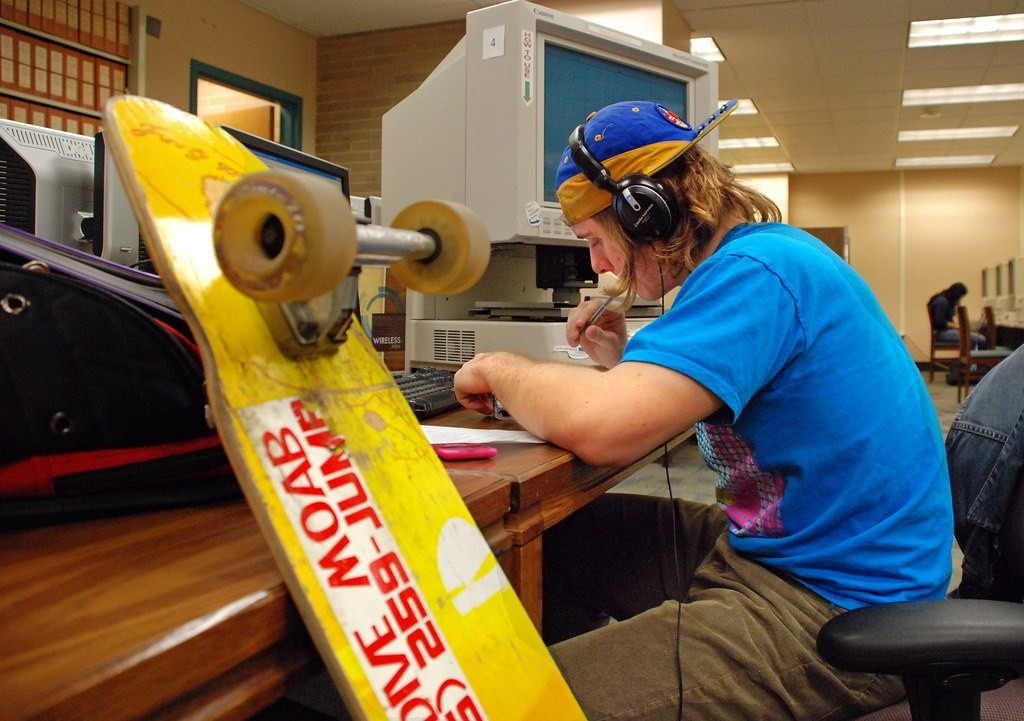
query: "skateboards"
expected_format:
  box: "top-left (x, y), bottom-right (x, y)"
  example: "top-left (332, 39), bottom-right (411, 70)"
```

top-left (95, 93), bottom-right (588, 721)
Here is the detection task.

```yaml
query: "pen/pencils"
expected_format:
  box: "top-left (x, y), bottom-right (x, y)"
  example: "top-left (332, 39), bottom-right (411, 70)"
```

top-left (578, 295), bottom-right (615, 335)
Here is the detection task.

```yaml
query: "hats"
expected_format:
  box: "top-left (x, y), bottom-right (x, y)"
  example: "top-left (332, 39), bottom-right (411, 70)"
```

top-left (556, 98), bottom-right (740, 227)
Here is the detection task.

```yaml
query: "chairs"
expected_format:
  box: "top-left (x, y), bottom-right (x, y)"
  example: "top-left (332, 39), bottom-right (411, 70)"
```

top-left (814, 344), bottom-right (1024, 721)
top-left (956, 305), bottom-right (1013, 403)
top-left (982, 305), bottom-right (1009, 350)
top-left (925, 304), bottom-right (975, 383)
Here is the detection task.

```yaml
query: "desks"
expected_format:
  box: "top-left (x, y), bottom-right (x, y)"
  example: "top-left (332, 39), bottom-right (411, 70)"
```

top-left (0, 467), bottom-right (514, 721)
top-left (410, 395), bottom-right (696, 640)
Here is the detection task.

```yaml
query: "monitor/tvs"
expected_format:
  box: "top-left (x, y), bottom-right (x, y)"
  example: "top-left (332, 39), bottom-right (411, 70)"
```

top-left (379, 0), bottom-right (720, 321)
top-left (0, 117), bottom-right (361, 335)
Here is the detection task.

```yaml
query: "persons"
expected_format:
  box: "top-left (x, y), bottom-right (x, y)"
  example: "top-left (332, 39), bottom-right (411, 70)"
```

top-left (927, 282), bottom-right (987, 374)
top-left (450, 98), bottom-right (957, 721)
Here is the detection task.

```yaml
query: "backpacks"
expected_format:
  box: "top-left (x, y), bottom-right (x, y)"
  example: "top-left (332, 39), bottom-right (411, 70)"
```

top-left (0, 222), bottom-right (245, 529)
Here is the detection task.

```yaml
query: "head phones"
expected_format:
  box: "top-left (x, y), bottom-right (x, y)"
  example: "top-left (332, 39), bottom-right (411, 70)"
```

top-left (569, 125), bottom-right (679, 244)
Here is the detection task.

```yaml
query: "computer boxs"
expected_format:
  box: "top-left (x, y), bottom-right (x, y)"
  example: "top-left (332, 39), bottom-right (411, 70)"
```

top-left (406, 319), bottom-right (659, 375)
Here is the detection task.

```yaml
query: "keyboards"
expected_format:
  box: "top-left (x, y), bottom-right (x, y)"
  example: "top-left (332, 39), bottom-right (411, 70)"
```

top-left (387, 365), bottom-right (464, 418)
